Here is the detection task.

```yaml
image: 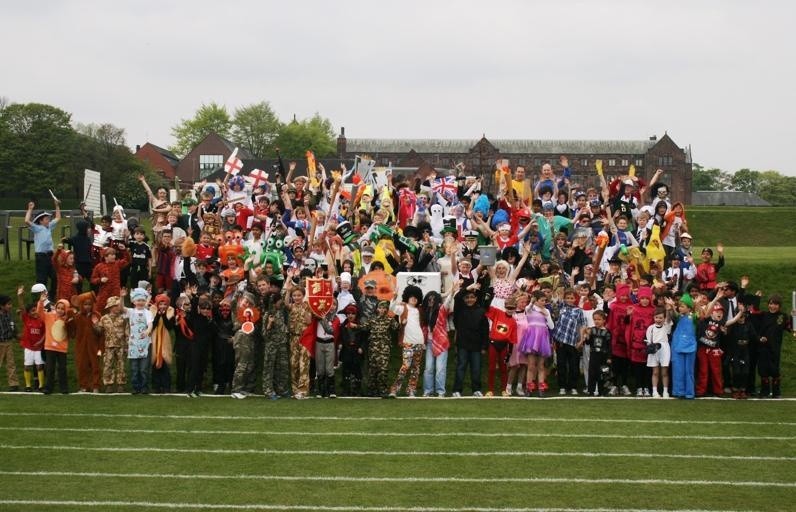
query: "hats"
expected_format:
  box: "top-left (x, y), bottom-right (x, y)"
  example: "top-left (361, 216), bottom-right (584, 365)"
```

top-left (606, 231), bottom-right (726, 311)
top-left (130, 288), bottom-right (148, 303)
top-left (516, 208), bottom-right (531, 219)
top-left (33, 210), bottom-right (53, 223)
top-left (155, 294), bottom-right (171, 307)
top-left (464, 230), bottom-right (479, 241)
top-left (182, 175), bottom-right (308, 306)
top-left (623, 179), bottom-right (634, 187)
top-left (104, 296), bottom-right (120, 310)
top-left (341, 184), bottom-right (457, 314)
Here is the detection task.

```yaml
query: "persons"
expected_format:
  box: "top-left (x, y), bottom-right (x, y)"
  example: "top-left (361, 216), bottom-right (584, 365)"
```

top-left (0, 150), bottom-right (796, 400)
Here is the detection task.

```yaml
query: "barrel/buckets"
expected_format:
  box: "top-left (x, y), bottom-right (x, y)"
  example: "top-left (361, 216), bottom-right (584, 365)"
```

top-left (478, 245), bottom-right (498, 266)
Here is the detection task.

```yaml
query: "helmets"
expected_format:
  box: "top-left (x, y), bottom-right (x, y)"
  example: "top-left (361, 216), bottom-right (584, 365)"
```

top-left (31, 283), bottom-right (50, 307)
top-left (573, 191), bottom-right (587, 201)
top-left (589, 199), bottom-right (601, 207)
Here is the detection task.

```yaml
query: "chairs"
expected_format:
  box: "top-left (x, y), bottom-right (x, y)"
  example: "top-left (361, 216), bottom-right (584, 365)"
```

top-left (124, 208), bottom-right (140, 224)
top-left (17, 226), bottom-right (34, 259)
top-left (61, 209), bottom-right (93, 251)
top-left (0, 212), bottom-right (12, 261)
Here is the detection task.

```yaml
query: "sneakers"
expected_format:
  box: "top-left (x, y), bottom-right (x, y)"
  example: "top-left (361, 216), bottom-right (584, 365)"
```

top-left (559, 385), bottom-right (781, 399)
top-left (9, 384), bottom-right (253, 399)
top-left (265, 382), bottom-right (548, 401)
